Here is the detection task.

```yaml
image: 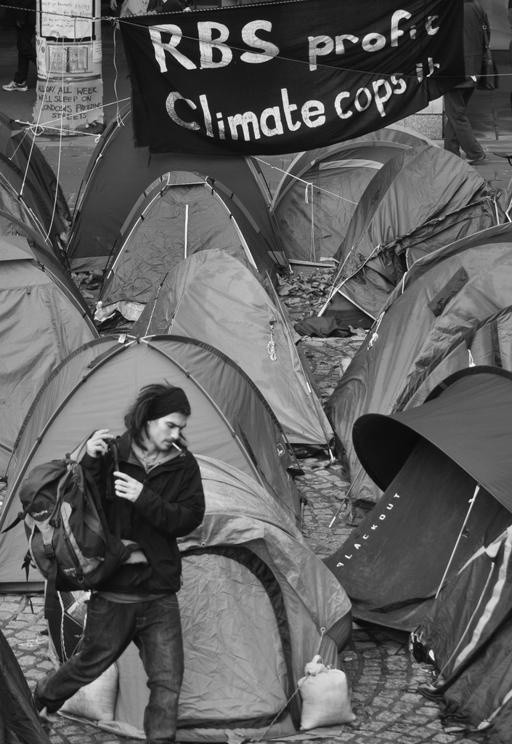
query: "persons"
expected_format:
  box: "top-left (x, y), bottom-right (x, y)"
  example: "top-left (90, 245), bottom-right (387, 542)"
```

top-left (444, 1), bottom-right (491, 165)
top-left (2, 0), bottom-right (36, 93)
top-left (33, 384), bottom-right (205, 744)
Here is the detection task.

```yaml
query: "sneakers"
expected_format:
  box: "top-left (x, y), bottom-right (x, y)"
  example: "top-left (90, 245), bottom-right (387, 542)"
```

top-left (464, 151), bottom-right (485, 164)
top-left (33, 681), bottom-right (58, 736)
top-left (2, 81), bottom-right (28, 93)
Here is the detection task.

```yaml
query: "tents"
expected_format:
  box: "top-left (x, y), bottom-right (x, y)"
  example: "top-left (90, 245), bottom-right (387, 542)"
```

top-left (61, 454), bottom-right (353, 743)
top-left (270, 124), bottom-right (510, 744)
top-left (1, 105), bottom-right (336, 586)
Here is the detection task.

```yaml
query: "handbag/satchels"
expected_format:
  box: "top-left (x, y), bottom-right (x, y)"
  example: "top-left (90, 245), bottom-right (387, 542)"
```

top-left (0, 428), bottom-right (157, 621)
top-left (476, 47), bottom-right (499, 91)
